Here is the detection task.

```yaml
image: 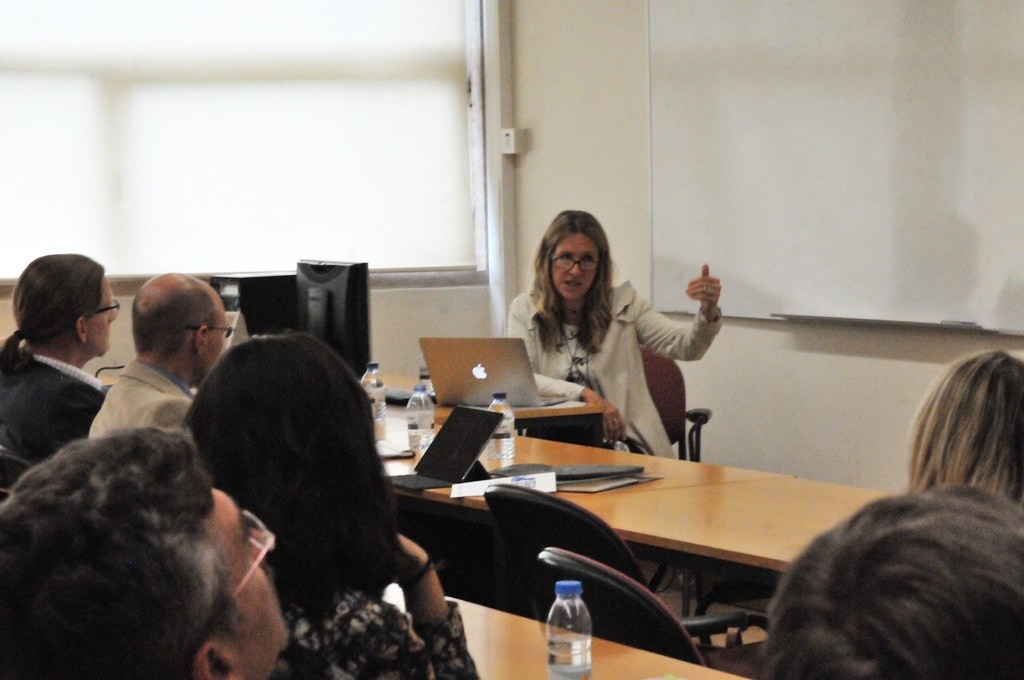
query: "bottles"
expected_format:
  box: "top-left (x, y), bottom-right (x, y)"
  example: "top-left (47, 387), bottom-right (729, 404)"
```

top-left (420, 354), bottom-right (434, 394)
top-left (406, 386), bottom-right (435, 455)
top-left (486, 392), bottom-right (516, 459)
top-left (360, 362), bottom-right (385, 422)
top-left (545, 580), bottom-right (592, 680)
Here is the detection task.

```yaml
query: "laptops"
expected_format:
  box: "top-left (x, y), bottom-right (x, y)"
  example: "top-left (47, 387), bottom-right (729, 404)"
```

top-left (418, 337), bottom-right (569, 407)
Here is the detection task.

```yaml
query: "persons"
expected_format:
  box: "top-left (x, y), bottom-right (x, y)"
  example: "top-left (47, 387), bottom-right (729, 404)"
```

top-left (906, 349), bottom-right (1024, 506)
top-left (179, 332), bottom-right (480, 680)
top-left (0, 254), bottom-right (120, 466)
top-left (757, 486), bottom-right (1024, 680)
top-left (506, 210), bottom-right (723, 459)
top-left (0, 425), bottom-right (286, 680)
top-left (89, 274), bottom-right (234, 440)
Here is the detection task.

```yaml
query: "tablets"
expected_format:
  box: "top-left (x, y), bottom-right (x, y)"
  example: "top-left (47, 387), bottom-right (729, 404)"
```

top-left (414, 406), bottom-right (506, 483)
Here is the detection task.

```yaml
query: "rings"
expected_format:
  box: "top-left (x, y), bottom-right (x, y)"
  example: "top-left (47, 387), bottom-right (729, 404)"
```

top-left (614, 419), bottom-right (619, 424)
top-left (705, 285), bottom-right (714, 296)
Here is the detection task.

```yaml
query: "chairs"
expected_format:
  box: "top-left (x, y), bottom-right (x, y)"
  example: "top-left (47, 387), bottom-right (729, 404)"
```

top-left (484, 483), bottom-right (690, 621)
top-left (638, 343), bottom-right (712, 464)
top-left (538, 545), bottom-right (772, 680)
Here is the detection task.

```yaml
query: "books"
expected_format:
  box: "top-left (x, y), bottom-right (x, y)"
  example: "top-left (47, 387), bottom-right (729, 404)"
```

top-left (375, 441), bottom-right (416, 459)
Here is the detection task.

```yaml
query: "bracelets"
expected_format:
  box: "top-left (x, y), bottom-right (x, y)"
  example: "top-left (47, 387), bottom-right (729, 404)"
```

top-left (402, 553), bottom-right (431, 588)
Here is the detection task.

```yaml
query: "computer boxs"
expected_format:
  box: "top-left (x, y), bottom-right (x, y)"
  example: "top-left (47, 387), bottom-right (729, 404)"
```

top-left (211, 272), bottom-right (296, 336)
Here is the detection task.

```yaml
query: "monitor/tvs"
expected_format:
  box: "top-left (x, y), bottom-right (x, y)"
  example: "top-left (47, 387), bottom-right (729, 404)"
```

top-left (295, 258), bottom-right (371, 381)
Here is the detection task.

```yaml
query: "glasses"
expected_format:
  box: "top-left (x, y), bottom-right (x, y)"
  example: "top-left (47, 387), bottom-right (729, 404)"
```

top-left (549, 255), bottom-right (601, 271)
top-left (65, 299), bottom-right (120, 329)
top-left (181, 509), bottom-right (276, 680)
top-left (186, 325), bottom-right (234, 338)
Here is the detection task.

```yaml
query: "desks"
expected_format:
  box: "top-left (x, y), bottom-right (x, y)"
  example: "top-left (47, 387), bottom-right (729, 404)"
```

top-left (365, 373), bottom-right (888, 680)
